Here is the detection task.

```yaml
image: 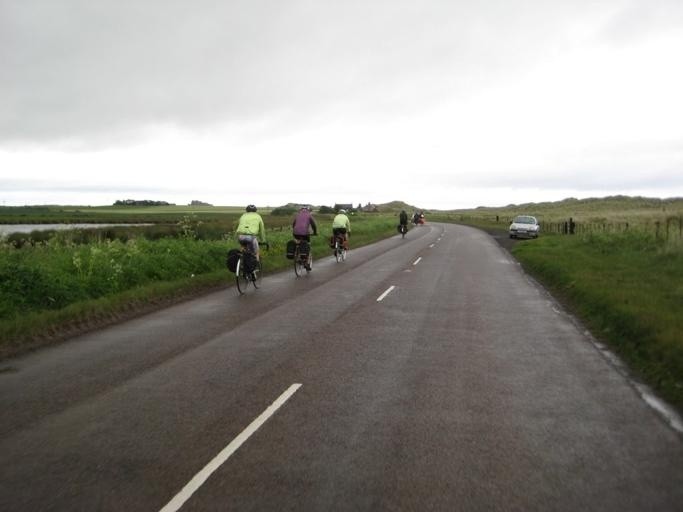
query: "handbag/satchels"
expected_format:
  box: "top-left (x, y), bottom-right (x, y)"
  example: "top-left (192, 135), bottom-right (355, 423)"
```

top-left (286, 240), bottom-right (310, 259)
top-left (329, 235), bottom-right (349, 250)
top-left (398, 224), bottom-right (408, 233)
top-left (227, 249), bottom-right (257, 272)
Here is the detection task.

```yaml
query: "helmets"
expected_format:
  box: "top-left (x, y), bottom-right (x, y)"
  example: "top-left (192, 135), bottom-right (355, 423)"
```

top-left (301, 205), bottom-right (310, 210)
top-left (245, 205), bottom-right (257, 212)
top-left (339, 209), bottom-right (346, 214)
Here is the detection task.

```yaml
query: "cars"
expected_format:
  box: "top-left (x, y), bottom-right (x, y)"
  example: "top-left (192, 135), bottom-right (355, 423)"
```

top-left (508, 215), bottom-right (540, 238)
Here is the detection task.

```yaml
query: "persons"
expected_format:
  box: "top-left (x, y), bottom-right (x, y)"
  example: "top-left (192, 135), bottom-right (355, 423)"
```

top-left (234, 205), bottom-right (265, 282)
top-left (414, 211), bottom-right (424, 224)
top-left (569, 217), bottom-right (575, 234)
top-left (399, 209), bottom-right (407, 225)
top-left (291, 204), bottom-right (317, 271)
top-left (332, 208), bottom-right (351, 256)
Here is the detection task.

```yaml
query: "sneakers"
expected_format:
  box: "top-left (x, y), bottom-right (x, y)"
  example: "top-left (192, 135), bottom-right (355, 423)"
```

top-left (306, 267), bottom-right (311, 270)
top-left (251, 271), bottom-right (256, 281)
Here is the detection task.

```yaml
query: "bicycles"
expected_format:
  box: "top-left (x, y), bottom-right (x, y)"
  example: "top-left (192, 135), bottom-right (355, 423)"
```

top-left (401, 225), bottom-right (405, 238)
top-left (334, 230), bottom-right (351, 263)
top-left (235, 241), bottom-right (270, 295)
top-left (293, 234), bottom-right (319, 278)
